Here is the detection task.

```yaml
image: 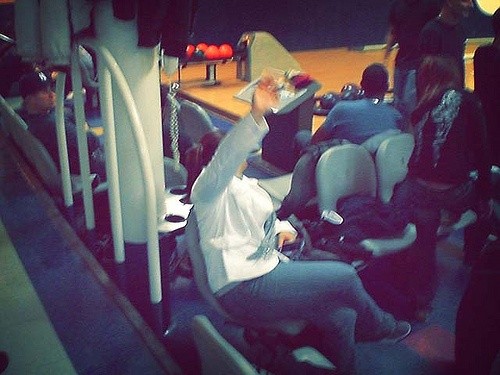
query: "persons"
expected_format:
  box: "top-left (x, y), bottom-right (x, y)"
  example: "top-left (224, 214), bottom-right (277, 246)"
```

top-left (17, 66), bottom-right (105, 175)
top-left (413, 0), bottom-right (473, 90)
top-left (187, 69), bottom-right (411, 375)
top-left (406, 53), bottom-right (494, 321)
top-left (382, 0), bottom-right (444, 106)
top-left (431, 227), bottom-right (500, 375)
top-left (474, 8), bottom-right (500, 171)
top-left (293, 62), bottom-right (404, 152)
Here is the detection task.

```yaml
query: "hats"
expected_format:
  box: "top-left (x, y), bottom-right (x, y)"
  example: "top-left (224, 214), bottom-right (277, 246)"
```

top-left (18, 70), bottom-right (57, 96)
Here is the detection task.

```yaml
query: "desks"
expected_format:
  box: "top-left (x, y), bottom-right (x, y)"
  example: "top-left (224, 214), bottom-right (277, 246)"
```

top-left (178, 54), bottom-right (243, 87)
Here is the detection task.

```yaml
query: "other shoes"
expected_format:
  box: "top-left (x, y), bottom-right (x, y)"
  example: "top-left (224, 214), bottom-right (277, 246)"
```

top-left (380, 321), bottom-right (412, 342)
top-left (274, 206), bottom-right (292, 220)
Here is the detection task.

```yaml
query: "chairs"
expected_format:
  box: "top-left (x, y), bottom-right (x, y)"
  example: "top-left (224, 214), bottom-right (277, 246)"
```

top-left (161, 126), bottom-right (479, 375)
top-left (0, 94), bottom-right (107, 207)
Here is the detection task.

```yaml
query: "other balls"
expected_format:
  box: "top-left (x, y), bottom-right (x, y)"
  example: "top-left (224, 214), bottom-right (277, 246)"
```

top-left (197, 43), bottom-right (208, 52)
top-left (186, 45), bottom-right (195, 56)
top-left (219, 44), bottom-right (233, 59)
top-left (341, 83), bottom-right (358, 100)
top-left (204, 45), bottom-right (219, 60)
top-left (320, 93), bottom-right (336, 109)
top-left (356, 88), bottom-right (364, 99)
